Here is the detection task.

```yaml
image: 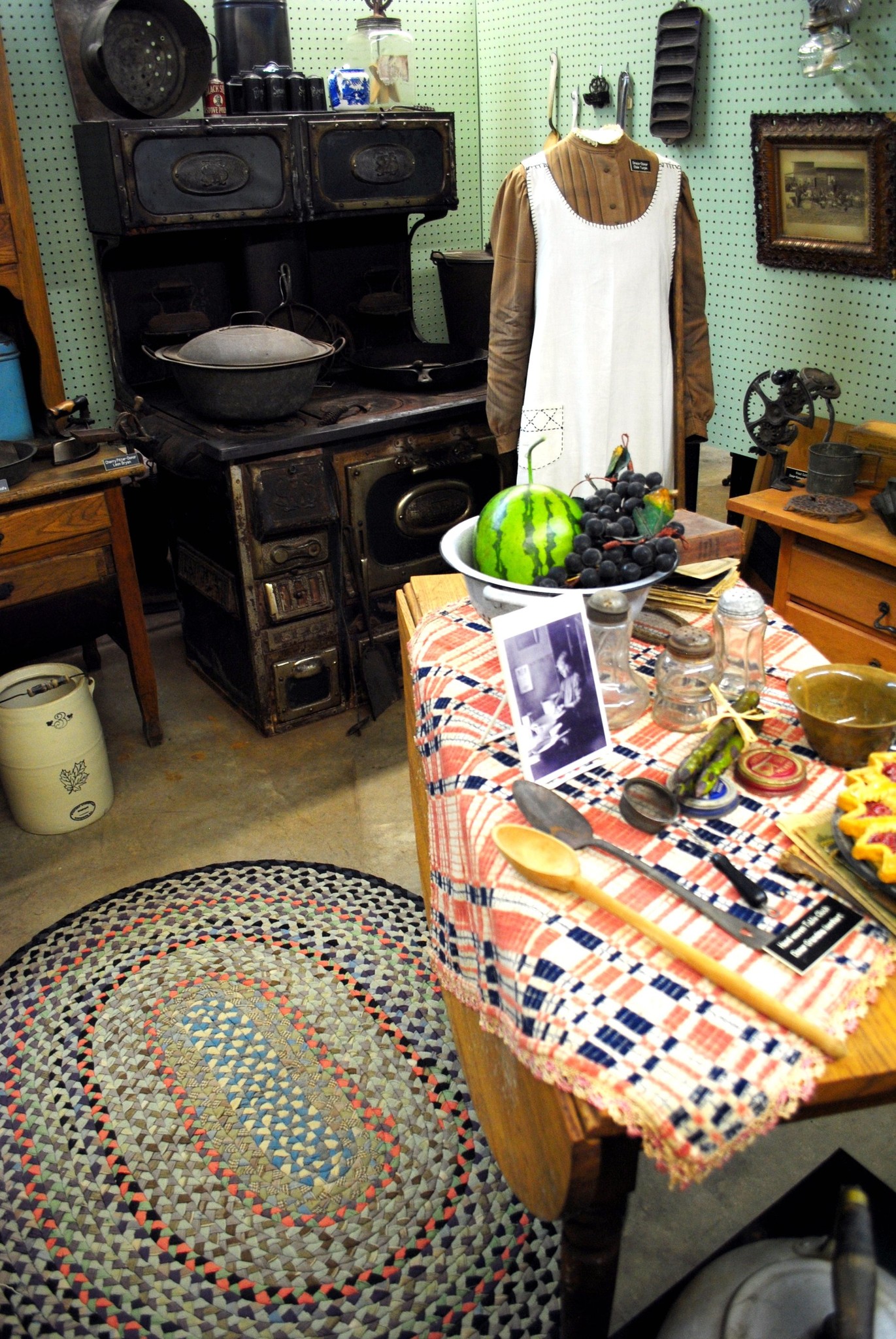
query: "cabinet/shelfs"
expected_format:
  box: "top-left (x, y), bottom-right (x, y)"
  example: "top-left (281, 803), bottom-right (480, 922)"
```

top-left (71, 109), bottom-right (458, 236)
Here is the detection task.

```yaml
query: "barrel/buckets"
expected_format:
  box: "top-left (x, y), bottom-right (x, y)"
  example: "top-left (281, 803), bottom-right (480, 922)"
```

top-left (0, 663), bottom-right (115, 835)
top-left (0, 337), bottom-right (34, 439)
top-left (212, 1), bottom-right (295, 115)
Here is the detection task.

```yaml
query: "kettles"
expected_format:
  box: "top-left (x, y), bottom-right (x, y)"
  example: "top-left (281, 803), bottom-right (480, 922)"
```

top-left (656, 1185), bottom-right (895, 1338)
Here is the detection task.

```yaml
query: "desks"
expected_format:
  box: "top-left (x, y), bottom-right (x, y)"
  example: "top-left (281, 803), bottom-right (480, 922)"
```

top-left (0, 443), bottom-right (165, 751)
top-left (723, 477), bottom-right (896, 672)
top-left (395, 573), bottom-right (896, 1339)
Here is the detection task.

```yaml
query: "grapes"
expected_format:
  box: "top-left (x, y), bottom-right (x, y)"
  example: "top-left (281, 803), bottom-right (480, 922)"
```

top-left (534, 469), bottom-right (685, 589)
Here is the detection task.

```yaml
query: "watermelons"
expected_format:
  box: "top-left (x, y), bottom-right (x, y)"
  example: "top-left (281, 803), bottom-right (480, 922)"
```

top-left (473, 482), bottom-right (585, 583)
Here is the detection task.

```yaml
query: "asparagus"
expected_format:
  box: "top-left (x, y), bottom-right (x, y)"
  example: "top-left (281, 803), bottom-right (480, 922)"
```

top-left (672, 692), bottom-right (765, 796)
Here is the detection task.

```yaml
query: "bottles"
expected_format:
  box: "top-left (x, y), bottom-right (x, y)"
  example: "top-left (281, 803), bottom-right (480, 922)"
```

top-left (350, 15), bottom-right (415, 109)
top-left (714, 588), bottom-right (766, 700)
top-left (583, 590), bottom-right (650, 727)
top-left (202, 72), bottom-right (329, 119)
top-left (652, 625), bottom-right (720, 733)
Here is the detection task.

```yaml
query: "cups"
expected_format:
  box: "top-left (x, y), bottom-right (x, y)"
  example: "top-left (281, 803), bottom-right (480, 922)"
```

top-left (786, 663), bottom-right (896, 769)
top-left (805, 442), bottom-right (882, 497)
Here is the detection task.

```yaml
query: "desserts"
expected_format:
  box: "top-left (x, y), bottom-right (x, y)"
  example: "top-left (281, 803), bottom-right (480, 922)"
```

top-left (834, 749), bottom-right (895, 887)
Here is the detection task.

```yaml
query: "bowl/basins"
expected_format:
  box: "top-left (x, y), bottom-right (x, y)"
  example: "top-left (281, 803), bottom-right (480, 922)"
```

top-left (0, 441), bottom-right (37, 486)
top-left (440, 515), bottom-right (680, 643)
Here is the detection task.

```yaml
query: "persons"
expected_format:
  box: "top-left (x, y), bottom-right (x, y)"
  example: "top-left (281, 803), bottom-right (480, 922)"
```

top-left (549, 653), bottom-right (590, 723)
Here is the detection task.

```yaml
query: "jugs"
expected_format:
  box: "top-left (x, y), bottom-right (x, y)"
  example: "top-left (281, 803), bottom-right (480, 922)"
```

top-left (329, 69), bottom-right (370, 113)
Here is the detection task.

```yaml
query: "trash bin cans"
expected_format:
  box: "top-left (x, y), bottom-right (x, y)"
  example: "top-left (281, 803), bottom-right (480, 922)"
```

top-left (0, 663), bottom-right (114, 835)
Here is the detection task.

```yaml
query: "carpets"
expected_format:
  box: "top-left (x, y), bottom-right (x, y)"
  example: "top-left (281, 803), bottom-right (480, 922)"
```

top-left (0, 859), bottom-right (566, 1339)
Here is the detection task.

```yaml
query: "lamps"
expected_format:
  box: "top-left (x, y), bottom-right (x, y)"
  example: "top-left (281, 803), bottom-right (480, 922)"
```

top-left (798, 0), bottom-right (863, 79)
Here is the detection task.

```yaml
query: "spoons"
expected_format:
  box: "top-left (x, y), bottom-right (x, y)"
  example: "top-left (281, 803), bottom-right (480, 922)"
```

top-left (493, 822), bottom-right (848, 1060)
top-left (512, 778), bottom-right (781, 946)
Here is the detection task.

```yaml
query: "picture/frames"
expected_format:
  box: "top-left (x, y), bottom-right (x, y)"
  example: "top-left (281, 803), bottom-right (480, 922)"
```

top-left (749, 111), bottom-right (896, 282)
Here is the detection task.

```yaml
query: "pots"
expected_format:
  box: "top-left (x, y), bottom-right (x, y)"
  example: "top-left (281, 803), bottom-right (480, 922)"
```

top-left (139, 280), bottom-right (347, 418)
top-left (356, 245), bottom-right (495, 391)
